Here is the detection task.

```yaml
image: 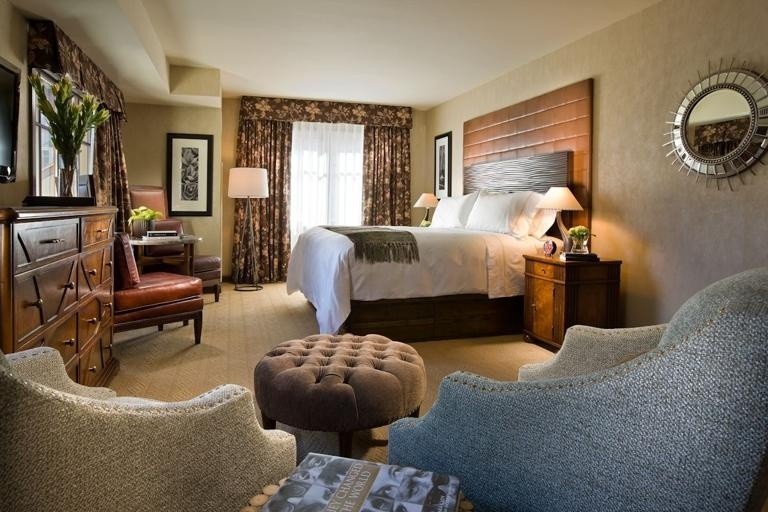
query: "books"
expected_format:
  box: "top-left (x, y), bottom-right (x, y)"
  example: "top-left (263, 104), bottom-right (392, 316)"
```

top-left (142, 231), bottom-right (180, 241)
top-left (560, 252), bottom-right (600, 262)
top-left (261, 451), bottom-right (460, 512)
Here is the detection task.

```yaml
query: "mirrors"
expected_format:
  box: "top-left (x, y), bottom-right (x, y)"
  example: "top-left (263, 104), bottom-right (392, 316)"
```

top-left (662, 56), bottom-right (768, 191)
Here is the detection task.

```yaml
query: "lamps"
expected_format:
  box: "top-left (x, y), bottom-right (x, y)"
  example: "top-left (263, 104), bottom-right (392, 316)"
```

top-left (413, 193), bottom-right (439, 221)
top-left (227, 168), bottom-right (270, 291)
top-left (535, 187), bottom-right (584, 252)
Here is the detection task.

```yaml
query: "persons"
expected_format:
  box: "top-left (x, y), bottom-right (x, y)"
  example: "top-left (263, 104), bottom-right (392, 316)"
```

top-left (266, 454), bottom-right (458, 512)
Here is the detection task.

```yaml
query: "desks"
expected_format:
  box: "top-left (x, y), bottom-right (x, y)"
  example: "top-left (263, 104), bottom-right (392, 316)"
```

top-left (239, 452), bottom-right (473, 512)
top-left (129, 237), bottom-right (203, 327)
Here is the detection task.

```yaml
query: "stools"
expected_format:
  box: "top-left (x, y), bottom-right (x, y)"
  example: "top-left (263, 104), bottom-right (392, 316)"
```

top-left (254, 332), bottom-right (426, 466)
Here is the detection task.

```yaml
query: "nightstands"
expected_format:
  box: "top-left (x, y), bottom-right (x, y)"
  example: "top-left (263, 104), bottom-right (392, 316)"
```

top-left (523, 254), bottom-right (622, 353)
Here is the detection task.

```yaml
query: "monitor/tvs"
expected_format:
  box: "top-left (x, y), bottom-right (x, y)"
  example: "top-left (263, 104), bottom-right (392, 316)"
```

top-left (0, 55), bottom-right (22, 184)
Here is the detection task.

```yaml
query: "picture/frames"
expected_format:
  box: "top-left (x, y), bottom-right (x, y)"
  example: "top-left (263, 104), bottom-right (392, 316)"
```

top-left (166, 132), bottom-right (213, 216)
top-left (433, 131), bottom-right (452, 200)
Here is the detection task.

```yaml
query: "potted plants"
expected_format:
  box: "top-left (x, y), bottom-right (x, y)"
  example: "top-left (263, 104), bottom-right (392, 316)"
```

top-left (569, 225), bottom-right (590, 249)
top-left (129, 206), bottom-right (164, 236)
top-left (29, 71), bottom-right (111, 197)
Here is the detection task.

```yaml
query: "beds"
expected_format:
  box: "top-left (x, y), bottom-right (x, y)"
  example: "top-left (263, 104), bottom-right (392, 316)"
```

top-left (308, 224), bottom-right (563, 343)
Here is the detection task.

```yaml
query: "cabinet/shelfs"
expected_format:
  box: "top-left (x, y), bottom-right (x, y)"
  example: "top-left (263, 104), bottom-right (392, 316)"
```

top-left (0, 206), bottom-right (120, 389)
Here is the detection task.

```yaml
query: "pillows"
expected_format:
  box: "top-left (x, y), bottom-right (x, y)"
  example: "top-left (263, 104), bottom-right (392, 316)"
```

top-left (465, 191), bottom-right (555, 239)
top-left (429, 191), bottom-right (479, 229)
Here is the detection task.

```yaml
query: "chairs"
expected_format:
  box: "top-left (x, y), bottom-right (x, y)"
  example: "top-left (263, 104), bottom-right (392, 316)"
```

top-left (114, 231), bottom-right (204, 343)
top-left (0, 347), bottom-right (297, 512)
top-left (386, 268), bottom-right (768, 512)
top-left (131, 218), bottom-right (221, 302)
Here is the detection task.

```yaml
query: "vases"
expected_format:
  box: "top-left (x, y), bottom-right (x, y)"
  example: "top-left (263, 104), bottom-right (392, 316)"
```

top-left (57, 150), bottom-right (78, 197)
top-left (570, 234), bottom-right (588, 253)
